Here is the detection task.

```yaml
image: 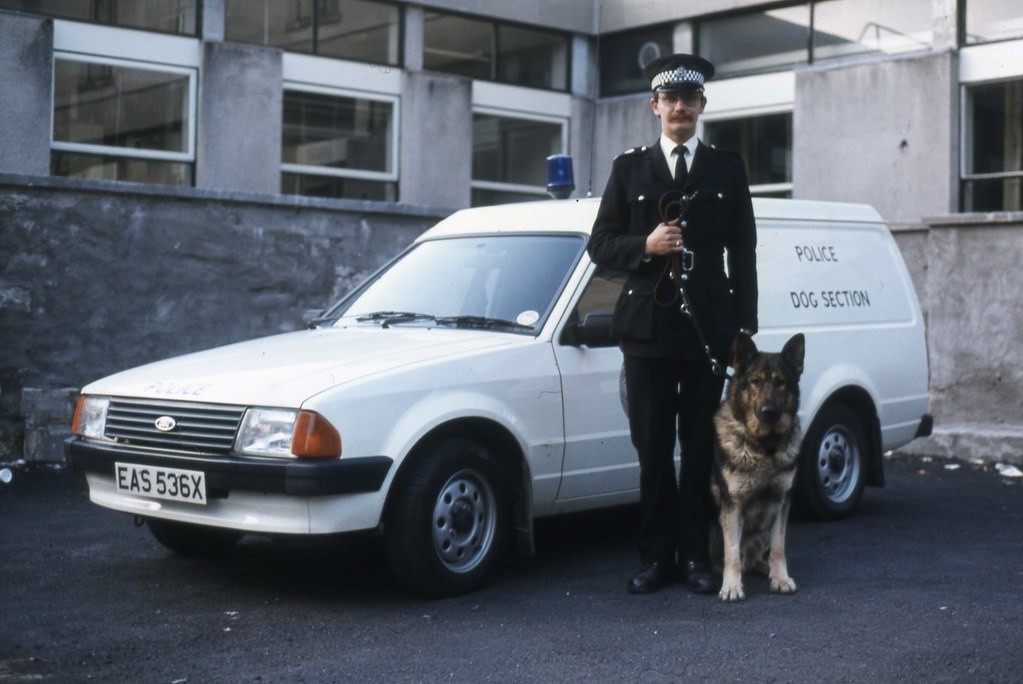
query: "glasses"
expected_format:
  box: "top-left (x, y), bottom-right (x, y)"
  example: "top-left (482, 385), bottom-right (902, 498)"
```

top-left (657, 94), bottom-right (700, 106)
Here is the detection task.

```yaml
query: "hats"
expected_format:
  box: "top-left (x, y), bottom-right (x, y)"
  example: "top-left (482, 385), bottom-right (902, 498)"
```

top-left (645, 54), bottom-right (715, 91)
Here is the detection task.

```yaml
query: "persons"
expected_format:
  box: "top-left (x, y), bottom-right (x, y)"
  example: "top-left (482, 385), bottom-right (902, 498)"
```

top-left (587, 53), bottom-right (758, 593)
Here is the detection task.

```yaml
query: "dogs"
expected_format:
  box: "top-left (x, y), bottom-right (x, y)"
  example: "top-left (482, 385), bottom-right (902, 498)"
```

top-left (704, 331), bottom-right (807, 601)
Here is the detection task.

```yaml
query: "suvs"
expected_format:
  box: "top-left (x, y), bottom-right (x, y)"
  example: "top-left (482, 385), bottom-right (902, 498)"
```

top-left (64, 193), bottom-right (935, 591)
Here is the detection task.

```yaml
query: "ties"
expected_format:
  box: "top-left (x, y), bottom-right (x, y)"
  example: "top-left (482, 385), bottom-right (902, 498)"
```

top-left (673, 145), bottom-right (688, 192)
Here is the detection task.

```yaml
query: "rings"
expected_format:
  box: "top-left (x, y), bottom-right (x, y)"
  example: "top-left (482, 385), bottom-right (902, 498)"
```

top-left (676, 240), bottom-right (679, 246)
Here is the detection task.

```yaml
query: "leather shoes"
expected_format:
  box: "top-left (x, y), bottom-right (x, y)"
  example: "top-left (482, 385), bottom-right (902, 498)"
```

top-left (678, 558), bottom-right (719, 593)
top-left (629, 561), bottom-right (677, 593)
